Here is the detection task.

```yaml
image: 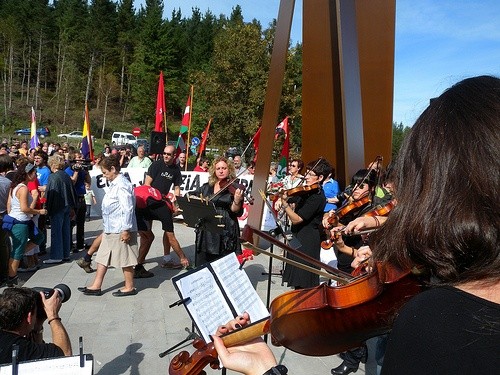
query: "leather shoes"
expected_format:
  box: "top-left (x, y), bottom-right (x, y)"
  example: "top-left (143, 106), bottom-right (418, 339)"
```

top-left (77, 287), bottom-right (101, 296)
top-left (112, 288), bottom-right (138, 296)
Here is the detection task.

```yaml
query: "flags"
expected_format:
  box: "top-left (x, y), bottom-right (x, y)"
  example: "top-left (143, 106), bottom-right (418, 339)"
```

top-left (30, 106), bottom-right (40, 150)
top-left (79, 100), bottom-right (96, 164)
top-left (155, 72), bottom-right (167, 132)
top-left (196, 118), bottom-right (212, 163)
top-left (175, 85), bottom-right (193, 157)
top-left (254, 116), bottom-right (291, 179)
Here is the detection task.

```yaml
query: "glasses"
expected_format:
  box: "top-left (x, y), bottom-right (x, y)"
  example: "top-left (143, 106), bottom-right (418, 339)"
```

top-left (305, 172), bottom-right (318, 177)
top-left (289, 164), bottom-right (297, 167)
top-left (351, 183), bottom-right (365, 189)
top-left (163, 152), bottom-right (171, 155)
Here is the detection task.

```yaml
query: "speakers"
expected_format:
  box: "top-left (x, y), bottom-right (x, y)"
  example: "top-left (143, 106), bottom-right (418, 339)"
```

top-left (150, 132), bottom-right (167, 155)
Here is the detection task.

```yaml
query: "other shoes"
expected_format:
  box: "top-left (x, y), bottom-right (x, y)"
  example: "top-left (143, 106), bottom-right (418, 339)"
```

top-left (18, 243), bottom-right (87, 272)
top-left (7, 276), bottom-right (24, 287)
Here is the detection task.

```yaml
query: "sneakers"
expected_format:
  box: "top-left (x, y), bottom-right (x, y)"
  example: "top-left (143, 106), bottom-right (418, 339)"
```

top-left (77, 257), bottom-right (94, 273)
top-left (162, 258), bottom-right (182, 268)
top-left (131, 267), bottom-right (154, 278)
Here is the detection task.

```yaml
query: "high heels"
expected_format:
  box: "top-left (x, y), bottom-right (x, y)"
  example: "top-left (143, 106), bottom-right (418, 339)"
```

top-left (331, 358), bottom-right (358, 375)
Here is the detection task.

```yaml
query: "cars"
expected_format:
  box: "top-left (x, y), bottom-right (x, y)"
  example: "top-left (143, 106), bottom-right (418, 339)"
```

top-left (57, 131), bottom-right (96, 143)
top-left (14, 126), bottom-right (51, 138)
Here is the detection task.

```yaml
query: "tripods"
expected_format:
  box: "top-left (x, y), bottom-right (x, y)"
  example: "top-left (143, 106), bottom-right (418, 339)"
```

top-left (159, 196), bottom-right (225, 359)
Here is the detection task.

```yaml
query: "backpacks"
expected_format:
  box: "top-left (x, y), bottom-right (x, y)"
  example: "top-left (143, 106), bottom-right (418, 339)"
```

top-left (133, 184), bottom-right (176, 214)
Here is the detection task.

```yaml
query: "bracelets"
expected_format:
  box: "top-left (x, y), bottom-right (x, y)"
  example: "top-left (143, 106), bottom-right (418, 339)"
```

top-left (48, 318), bottom-right (62, 324)
top-left (371, 215), bottom-right (380, 230)
top-left (262, 364), bottom-right (289, 375)
top-left (351, 246), bottom-right (354, 259)
top-left (32, 327), bottom-right (43, 335)
top-left (281, 203), bottom-right (289, 209)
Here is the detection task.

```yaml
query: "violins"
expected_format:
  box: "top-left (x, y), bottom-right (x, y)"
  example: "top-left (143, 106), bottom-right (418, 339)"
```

top-left (219, 177), bottom-right (255, 206)
top-left (169, 255), bottom-right (430, 375)
top-left (269, 183), bottom-right (321, 201)
top-left (321, 196), bottom-right (398, 250)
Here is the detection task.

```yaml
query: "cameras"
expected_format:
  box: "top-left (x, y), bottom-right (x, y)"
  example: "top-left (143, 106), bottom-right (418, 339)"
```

top-left (69, 159), bottom-right (93, 170)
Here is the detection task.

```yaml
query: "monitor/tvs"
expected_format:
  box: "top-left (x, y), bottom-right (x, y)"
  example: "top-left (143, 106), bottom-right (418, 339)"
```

top-left (32, 284), bottom-right (70, 323)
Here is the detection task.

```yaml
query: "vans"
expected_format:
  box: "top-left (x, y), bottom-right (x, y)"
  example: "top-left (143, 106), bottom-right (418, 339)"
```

top-left (111, 131), bottom-right (137, 146)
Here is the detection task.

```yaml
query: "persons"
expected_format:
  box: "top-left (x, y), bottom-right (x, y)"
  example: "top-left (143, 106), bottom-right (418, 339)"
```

top-left (142, 145), bottom-right (183, 268)
top-left (0, 139), bottom-right (152, 289)
top-left (186, 156), bottom-right (245, 271)
top-left (323, 169), bottom-right (379, 374)
top-left (177, 152), bottom-right (305, 181)
top-left (208, 76), bottom-right (500, 375)
top-left (0, 286), bottom-right (73, 366)
top-left (329, 214), bottom-right (390, 260)
top-left (277, 157), bottom-right (327, 291)
top-left (78, 185), bottom-right (192, 278)
top-left (320, 159), bottom-right (396, 213)
top-left (77, 158), bottom-right (139, 296)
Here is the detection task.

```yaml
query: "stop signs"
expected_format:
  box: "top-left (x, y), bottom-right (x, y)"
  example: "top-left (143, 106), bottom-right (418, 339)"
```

top-left (132, 128), bottom-right (140, 136)
